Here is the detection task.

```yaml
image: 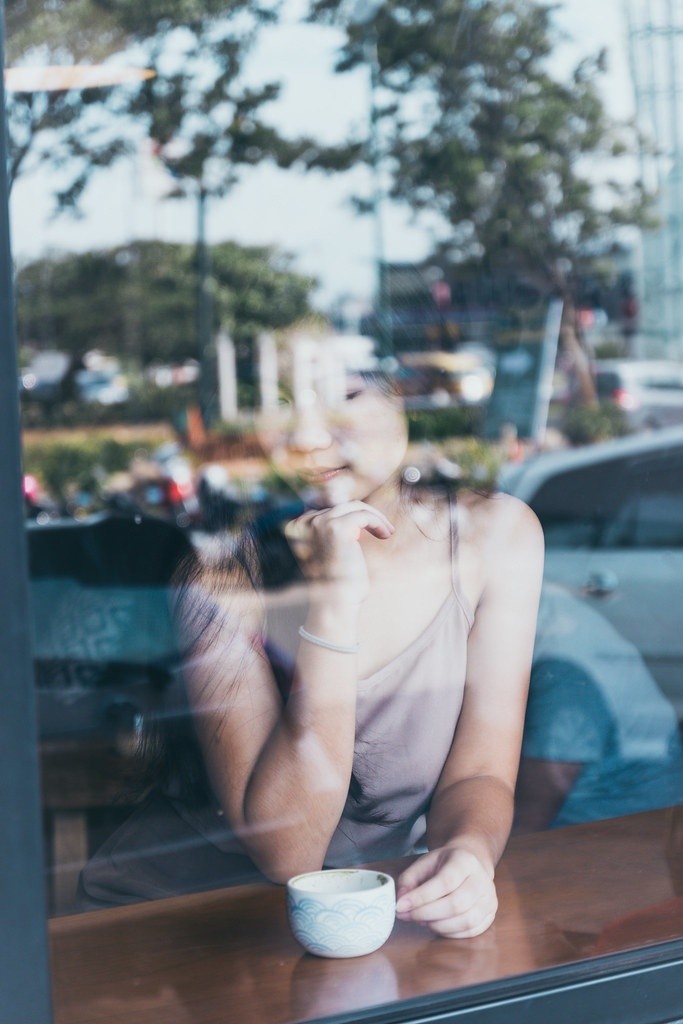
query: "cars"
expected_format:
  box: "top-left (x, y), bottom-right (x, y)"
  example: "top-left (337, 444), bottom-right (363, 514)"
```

top-left (390, 347), bottom-right (493, 408)
top-left (498, 424), bottom-right (682, 745)
top-left (25, 495), bottom-right (310, 799)
top-left (590, 354), bottom-right (683, 419)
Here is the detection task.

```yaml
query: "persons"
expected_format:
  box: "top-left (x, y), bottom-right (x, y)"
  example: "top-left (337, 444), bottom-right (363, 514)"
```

top-left (163, 264), bottom-right (548, 942)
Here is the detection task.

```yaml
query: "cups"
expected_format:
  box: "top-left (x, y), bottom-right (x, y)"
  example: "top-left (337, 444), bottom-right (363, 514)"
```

top-left (286, 868), bottom-right (395, 958)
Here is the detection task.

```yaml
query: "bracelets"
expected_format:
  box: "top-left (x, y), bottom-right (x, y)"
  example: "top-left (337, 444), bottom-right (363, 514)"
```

top-left (298, 623), bottom-right (368, 656)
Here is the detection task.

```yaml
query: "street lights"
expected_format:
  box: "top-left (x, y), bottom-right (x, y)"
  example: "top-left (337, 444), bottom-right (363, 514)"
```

top-left (163, 163), bottom-right (216, 432)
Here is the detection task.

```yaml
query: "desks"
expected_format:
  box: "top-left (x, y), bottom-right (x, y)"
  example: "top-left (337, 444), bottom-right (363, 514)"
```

top-left (49, 807), bottom-right (682, 1024)
